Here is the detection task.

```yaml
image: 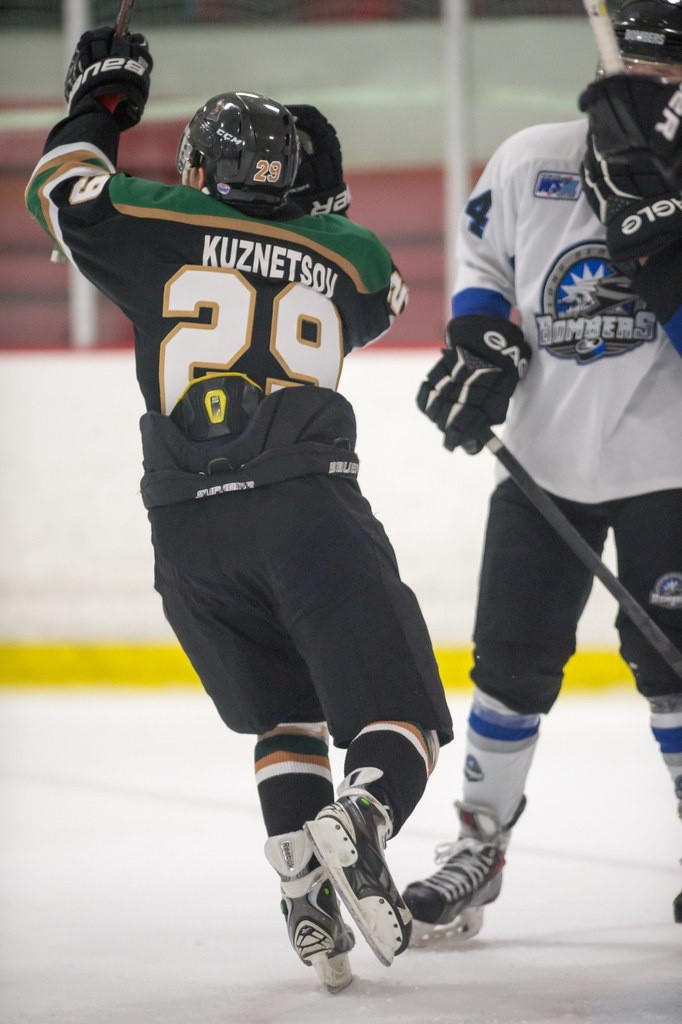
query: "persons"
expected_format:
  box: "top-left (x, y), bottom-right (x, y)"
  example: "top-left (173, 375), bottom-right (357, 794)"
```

top-left (398, 2), bottom-right (682, 938)
top-left (22, 23), bottom-right (454, 994)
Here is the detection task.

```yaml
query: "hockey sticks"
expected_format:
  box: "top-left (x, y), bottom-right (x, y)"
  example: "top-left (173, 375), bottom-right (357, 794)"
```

top-left (476, 427), bottom-right (682, 678)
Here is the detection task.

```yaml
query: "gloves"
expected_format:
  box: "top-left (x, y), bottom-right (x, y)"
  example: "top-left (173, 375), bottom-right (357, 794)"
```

top-left (581, 127), bottom-right (682, 263)
top-left (418, 314), bottom-right (533, 454)
top-left (284, 103), bottom-right (351, 215)
top-left (64, 27), bottom-right (152, 133)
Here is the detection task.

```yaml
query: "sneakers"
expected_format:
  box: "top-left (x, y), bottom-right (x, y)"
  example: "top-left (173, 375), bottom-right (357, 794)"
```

top-left (674, 774), bottom-right (682, 924)
top-left (301, 767), bottom-right (412, 967)
top-left (402, 792), bottom-right (527, 950)
top-left (264, 831), bottom-right (356, 995)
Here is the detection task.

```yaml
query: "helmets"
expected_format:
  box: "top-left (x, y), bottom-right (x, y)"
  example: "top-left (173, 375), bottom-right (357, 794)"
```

top-left (611, 0), bottom-right (682, 64)
top-left (184, 93), bottom-right (302, 201)
